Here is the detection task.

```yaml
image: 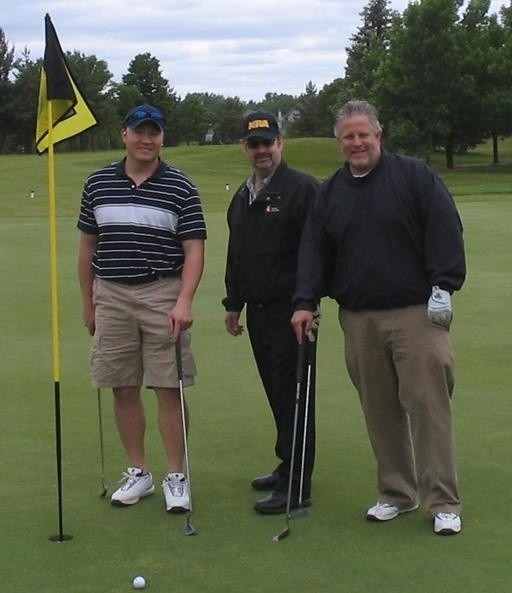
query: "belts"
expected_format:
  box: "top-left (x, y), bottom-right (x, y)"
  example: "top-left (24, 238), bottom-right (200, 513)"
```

top-left (112, 274), bottom-right (165, 285)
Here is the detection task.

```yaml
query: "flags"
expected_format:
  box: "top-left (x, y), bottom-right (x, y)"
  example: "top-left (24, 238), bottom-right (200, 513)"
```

top-left (32, 12), bottom-right (98, 156)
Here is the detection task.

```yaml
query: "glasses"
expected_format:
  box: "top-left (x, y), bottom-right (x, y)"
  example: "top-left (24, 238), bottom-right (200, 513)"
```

top-left (246, 138), bottom-right (274, 148)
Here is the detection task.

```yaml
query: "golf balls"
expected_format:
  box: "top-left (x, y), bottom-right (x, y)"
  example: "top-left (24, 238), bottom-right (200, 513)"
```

top-left (133, 576), bottom-right (146, 588)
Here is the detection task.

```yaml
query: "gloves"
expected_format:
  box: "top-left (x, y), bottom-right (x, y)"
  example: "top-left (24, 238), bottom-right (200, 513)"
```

top-left (427, 286), bottom-right (452, 326)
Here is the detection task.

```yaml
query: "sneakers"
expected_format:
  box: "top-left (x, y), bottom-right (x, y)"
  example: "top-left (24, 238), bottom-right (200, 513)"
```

top-left (254, 491), bottom-right (312, 514)
top-left (110, 467), bottom-right (155, 505)
top-left (367, 501), bottom-right (419, 521)
top-left (252, 472), bottom-right (280, 491)
top-left (433, 512), bottom-right (462, 535)
top-left (162, 472), bottom-right (190, 513)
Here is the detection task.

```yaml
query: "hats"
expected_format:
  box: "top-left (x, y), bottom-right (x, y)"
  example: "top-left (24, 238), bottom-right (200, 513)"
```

top-left (243, 111), bottom-right (279, 141)
top-left (122, 105), bottom-right (164, 133)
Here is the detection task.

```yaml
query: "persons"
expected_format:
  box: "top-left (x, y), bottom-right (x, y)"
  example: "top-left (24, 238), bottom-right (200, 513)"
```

top-left (221, 111), bottom-right (322, 513)
top-left (291, 98), bottom-right (468, 535)
top-left (78, 103), bottom-right (208, 514)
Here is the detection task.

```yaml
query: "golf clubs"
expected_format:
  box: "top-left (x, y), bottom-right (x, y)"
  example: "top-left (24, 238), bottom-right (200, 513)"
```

top-left (175, 331), bottom-right (196, 535)
top-left (97, 388), bottom-right (110, 500)
top-left (272, 321), bottom-right (318, 541)
